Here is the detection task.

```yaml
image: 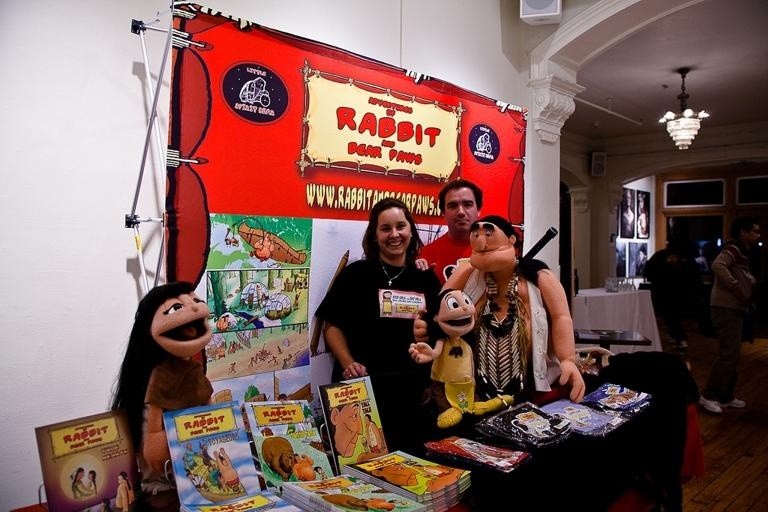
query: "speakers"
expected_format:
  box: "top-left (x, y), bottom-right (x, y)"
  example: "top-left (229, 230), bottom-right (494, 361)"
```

top-left (519, 0), bottom-right (562, 26)
top-left (591, 152), bottom-right (607, 177)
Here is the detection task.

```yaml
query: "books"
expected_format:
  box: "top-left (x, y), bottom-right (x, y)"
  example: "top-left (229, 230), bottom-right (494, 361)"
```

top-left (161, 377), bottom-right (472, 512)
top-left (34, 410), bottom-right (135, 512)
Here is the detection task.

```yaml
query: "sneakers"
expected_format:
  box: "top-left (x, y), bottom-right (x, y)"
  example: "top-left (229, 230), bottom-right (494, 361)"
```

top-left (699, 395), bottom-right (722, 412)
top-left (719, 398), bottom-right (746, 408)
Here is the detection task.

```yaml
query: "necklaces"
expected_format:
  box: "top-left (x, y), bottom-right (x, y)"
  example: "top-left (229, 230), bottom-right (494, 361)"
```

top-left (379, 257), bottom-right (408, 286)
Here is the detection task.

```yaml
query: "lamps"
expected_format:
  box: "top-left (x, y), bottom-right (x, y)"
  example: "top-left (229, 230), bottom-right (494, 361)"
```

top-left (659, 67), bottom-right (710, 151)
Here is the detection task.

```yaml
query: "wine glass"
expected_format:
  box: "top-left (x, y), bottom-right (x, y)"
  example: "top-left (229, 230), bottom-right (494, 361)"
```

top-left (605, 275), bottom-right (636, 291)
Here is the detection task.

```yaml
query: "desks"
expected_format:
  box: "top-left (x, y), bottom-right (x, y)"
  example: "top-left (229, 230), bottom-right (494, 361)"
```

top-left (574, 329), bottom-right (651, 353)
top-left (132, 351), bottom-right (699, 512)
top-left (571, 290), bottom-right (662, 355)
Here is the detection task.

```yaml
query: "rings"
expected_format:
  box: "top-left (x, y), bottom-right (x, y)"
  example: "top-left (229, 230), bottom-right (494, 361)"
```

top-left (348, 374), bottom-right (352, 378)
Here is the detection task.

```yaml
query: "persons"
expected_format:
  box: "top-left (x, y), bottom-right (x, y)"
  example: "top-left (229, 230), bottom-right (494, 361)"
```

top-left (413, 178), bottom-right (482, 288)
top-left (413, 216), bottom-right (586, 404)
top-left (616, 249), bottom-right (624, 276)
top-left (72, 468), bottom-right (92, 498)
top-left (620, 188), bottom-right (635, 238)
top-left (409, 289), bottom-right (514, 429)
top-left (637, 191), bottom-right (650, 238)
top-left (110, 281), bottom-right (214, 494)
top-left (697, 216), bottom-right (761, 414)
top-left (644, 242), bottom-right (723, 350)
top-left (635, 243), bottom-right (647, 276)
top-left (314, 198), bottom-right (444, 456)
